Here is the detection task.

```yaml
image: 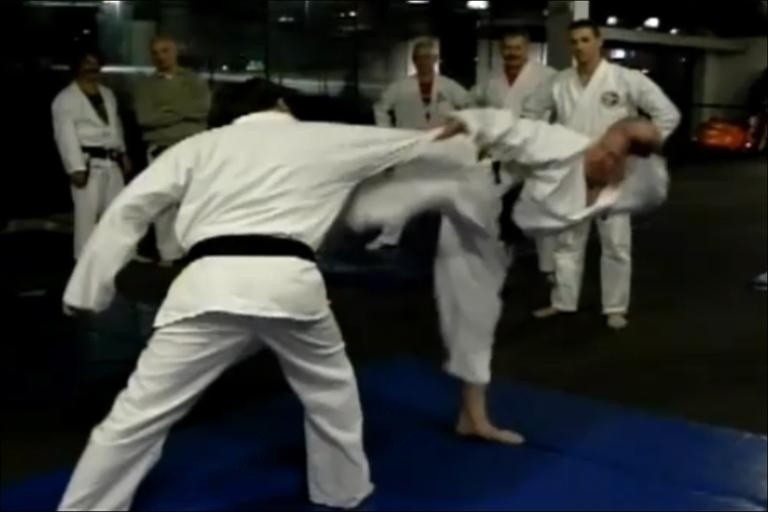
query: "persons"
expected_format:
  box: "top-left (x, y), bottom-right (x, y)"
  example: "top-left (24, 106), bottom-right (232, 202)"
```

top-left (50, 51), bottom-right (130, 263)
top-left (466, 27), bottom-right (563, 125)
top-left (363, 39), bottom-right (474, 253)
top-left (57, 77), bottom-right (454, 512)
top-left (133, 33), bottom-right (211, 276)
top-left (328, 106), bottom-right (670, 448)
top-left (525, 19), bottom-right (682, 329)
